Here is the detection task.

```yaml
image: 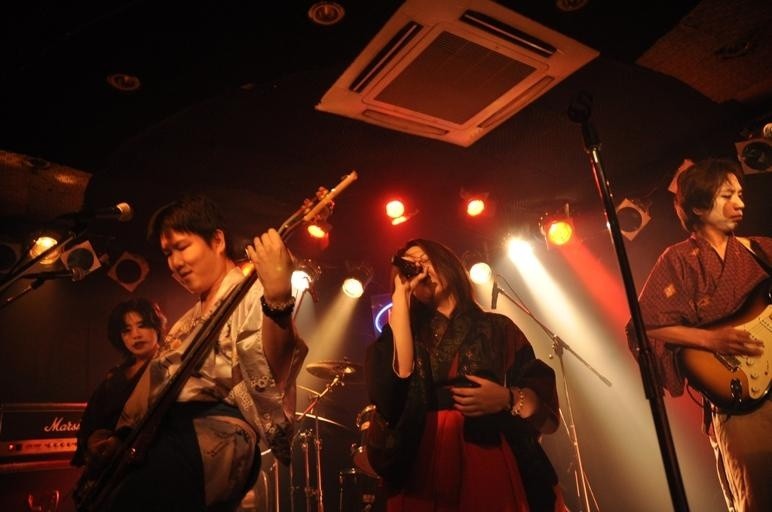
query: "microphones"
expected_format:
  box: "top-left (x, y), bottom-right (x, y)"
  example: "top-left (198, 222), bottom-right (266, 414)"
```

top-left (391, 255), bottom-right (423, 278)
top-left (491, 283), bottom-right (497, 309)
top-left (763, 123), bottom-right (772, 139)
top-left (74, 201), bottom-right (133, 227)
top-left (21, 266), bottom-right (86, 282)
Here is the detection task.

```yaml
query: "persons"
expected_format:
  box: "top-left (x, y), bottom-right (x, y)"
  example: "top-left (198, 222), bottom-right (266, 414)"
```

top-left (72, 198), bottom-right (561, 512)
top-left (625, 161), bottom-right (772, 512)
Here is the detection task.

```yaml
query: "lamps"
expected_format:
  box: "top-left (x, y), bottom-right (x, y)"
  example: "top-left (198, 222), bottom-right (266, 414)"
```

top-left (291, 257), bottom-right (322, 292)
top-left (460, 250), bottom-right (492, 285)
top-left (307, 222), bottom-right (334, 238)
top-left (341, 258), bottom-right (376, 298)
top-left (26, 229), bottom-right (64, 265)
top-left (536, 210), bottom-right (575, 250)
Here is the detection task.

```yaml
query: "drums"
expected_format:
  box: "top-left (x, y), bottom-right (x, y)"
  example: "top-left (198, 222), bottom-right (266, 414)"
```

top-left (350, 405), bottom-right (383, 480)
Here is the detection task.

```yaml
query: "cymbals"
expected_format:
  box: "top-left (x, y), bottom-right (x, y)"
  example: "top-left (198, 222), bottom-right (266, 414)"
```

top-left (306, 361), bottom-right (377, 382)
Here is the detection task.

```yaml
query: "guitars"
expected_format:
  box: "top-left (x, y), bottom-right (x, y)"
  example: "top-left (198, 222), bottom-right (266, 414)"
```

top-left (296, 412), bottom-right (348, 429)
top-left (68, 172), bottom-right (358, 507)
top-left (674, 274), bottom-right (771, 416)
